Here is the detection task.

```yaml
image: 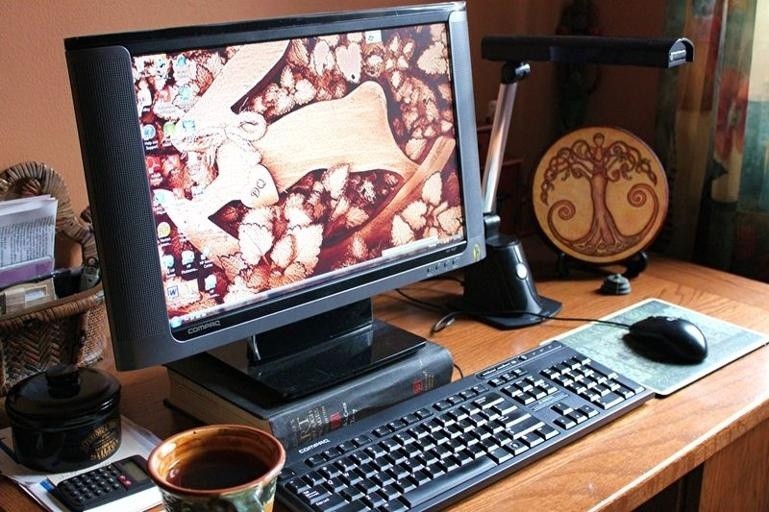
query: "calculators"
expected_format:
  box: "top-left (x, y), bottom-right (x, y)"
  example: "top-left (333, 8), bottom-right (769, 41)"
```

top-left (56, 455), bottom-right (157, 512)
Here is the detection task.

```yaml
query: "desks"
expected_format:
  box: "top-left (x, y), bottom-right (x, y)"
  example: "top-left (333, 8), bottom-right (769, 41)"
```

top-left (1, 253), bottom-right (769, 512)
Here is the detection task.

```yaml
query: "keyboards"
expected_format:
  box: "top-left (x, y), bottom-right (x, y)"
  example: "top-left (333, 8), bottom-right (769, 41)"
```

top-left (275, 339), bottom-right (655, 511)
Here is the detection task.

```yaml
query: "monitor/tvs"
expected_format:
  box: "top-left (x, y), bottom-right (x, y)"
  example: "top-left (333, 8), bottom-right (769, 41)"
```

top-left (63, 1), bottom-right (490, 409)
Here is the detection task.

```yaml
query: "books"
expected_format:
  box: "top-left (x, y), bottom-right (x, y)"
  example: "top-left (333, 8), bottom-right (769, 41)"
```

top-left (0, 253), bottom-right (55, 290)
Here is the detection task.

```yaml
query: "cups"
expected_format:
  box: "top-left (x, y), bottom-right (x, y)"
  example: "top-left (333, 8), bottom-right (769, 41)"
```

top-left (147, 423), bottom-right (289, 512)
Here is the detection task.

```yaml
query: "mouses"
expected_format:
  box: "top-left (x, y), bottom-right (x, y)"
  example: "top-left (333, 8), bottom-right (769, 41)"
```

top-left (628, 312), bottom-right (710, 361)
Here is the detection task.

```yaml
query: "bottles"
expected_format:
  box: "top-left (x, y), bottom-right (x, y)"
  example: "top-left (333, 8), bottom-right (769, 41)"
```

top-left (4, 361), bottom-right (120, 477)
